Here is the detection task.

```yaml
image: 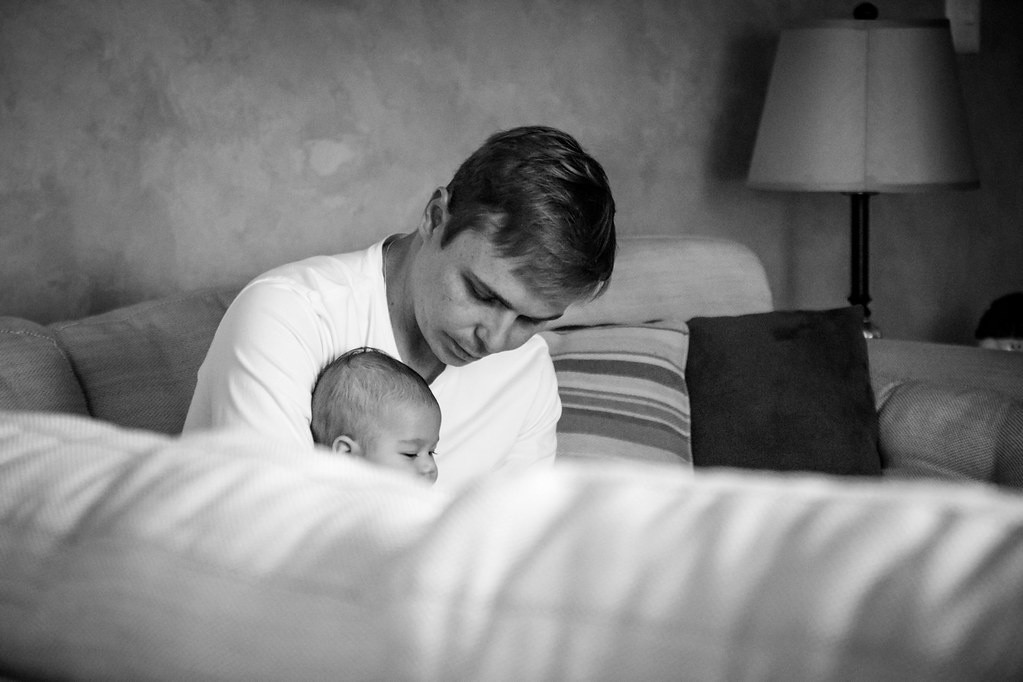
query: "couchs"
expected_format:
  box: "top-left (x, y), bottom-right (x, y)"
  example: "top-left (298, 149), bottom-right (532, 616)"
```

top-left (0, 231), bottom-right (1023, 682)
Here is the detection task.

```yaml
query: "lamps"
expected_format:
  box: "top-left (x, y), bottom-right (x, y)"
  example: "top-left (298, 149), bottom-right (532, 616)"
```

top-left (744, 3), bottom-right (981, 339)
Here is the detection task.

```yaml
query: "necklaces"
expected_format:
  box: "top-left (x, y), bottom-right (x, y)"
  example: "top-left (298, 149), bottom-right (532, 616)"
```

top-left (385, 239), bottom-right (400, 285)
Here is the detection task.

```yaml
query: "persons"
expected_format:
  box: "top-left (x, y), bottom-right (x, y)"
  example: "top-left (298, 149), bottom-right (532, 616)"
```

top-left (182, 126), bottom-right (616, 467)
top-left (310, 346), bottom-right (441, 481)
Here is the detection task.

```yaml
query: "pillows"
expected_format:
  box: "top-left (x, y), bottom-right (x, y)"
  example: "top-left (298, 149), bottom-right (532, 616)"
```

top-left (685, 303), bottom-right (883, 476)
top-left (542, 317), bottom-right (694, 468)
